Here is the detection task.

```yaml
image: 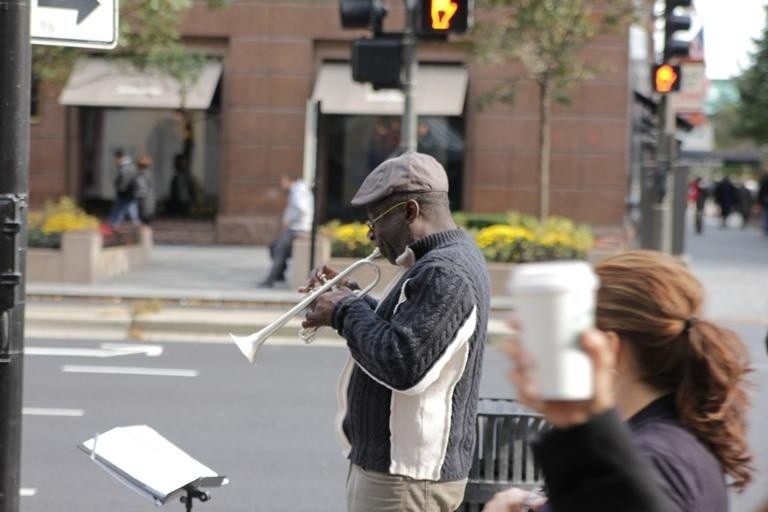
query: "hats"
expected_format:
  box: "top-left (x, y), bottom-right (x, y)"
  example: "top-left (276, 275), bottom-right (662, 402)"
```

top-left (351, 151), bottom-right (450, 203)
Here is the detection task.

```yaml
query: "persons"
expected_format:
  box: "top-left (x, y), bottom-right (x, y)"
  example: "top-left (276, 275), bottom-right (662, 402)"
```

top-left (298, 149), bottom-right (492, 512)
top-left (164, 154), bottom-right (204, 216)
top-left (133, 155), bottom-right (157, 247)
top-left (107, 148), bottom-right (139, 228)
top-left (479, 248), bottom-right (759, 512)
top-left (497, 307), bottom-right (682, 512)
top-left (689, 170), bottom-right (767, 240)
top-left (254, 170), bottom-right (315, 289)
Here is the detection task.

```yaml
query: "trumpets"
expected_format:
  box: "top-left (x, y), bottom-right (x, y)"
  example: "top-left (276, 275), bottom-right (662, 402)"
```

top-left (229, 247), bottom-right (381, 365)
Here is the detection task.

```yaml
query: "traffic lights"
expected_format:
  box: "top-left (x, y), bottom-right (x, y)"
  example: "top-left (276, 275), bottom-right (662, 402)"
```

top-left (339, 0), bottom-right (384, 39)
top-left (650, 64), bottom-right (680, 93)
top-left (414, 0), bottom-right (472, 39)
top-left (661, 0), bottom-right (692, 60)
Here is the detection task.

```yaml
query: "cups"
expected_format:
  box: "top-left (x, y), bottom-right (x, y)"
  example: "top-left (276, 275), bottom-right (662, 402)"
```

top-left (507, 260), bottom-right (603, 403)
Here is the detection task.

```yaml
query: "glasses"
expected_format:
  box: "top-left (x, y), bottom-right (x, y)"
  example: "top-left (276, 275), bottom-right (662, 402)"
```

top-left (364, 201), bottom-right (412, 231)
